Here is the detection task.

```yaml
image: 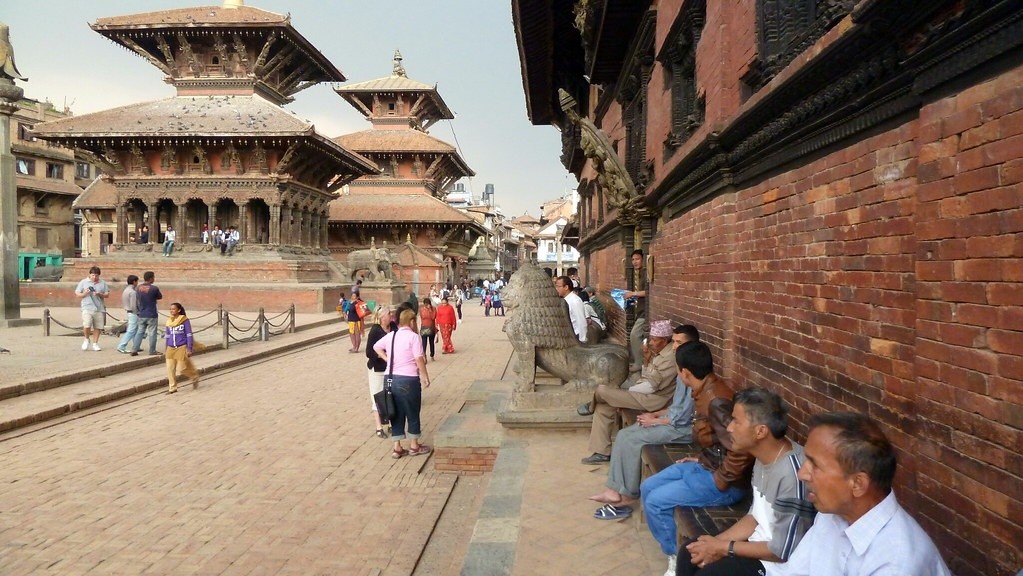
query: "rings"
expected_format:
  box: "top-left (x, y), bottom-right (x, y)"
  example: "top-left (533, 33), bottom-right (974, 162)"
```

top-left (702, 562), bottom-right (708, 567)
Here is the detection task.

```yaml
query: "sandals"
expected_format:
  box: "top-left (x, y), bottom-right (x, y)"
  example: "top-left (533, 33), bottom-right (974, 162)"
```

top-left (409, 444), bottom-right (430, 456)
top-left (391, 447), bottom-right (409, 459)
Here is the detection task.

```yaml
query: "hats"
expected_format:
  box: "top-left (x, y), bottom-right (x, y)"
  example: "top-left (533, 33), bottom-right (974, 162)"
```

top-left (581, 286), bottom-right (595, 293)
top-left (649, 319), bottom-right (673, 337)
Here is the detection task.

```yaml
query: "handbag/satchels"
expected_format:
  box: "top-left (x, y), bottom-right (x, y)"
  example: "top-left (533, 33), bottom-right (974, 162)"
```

top-left (420, 326), bottom-right (433, 336)
top-left (374, 386), bottom-right (394, 421)
top-left (355, 299), bottom-right (372, 319)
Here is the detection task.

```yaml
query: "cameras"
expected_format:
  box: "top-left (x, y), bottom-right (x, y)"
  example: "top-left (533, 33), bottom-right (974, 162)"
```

top-left (88, 286), bottom-right (95, 293)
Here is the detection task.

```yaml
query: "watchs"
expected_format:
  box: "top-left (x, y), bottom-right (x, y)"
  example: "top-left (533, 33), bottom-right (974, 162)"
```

top-left (728, 541), bottom-right (735, 559)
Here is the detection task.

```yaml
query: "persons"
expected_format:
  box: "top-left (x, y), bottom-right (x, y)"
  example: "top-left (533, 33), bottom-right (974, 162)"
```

top-left (767, 411), bottom-right (953, 576)
top-left (373, 303), bottom-right (430, 458)
top-left (676, 388), bottom-right (818, 576)
top-left (436, 298), bottom-right (456, 354)
top-left (556, 276), bottom-right (607, 344)
top-left (164, 303), bottom-right (199, 392)
top-left (337, 279), bottom-right (362, 352)
top-left (129, 225), bottom-right (148, 243)
top-left (163, 225), bottom-right (175, 257)
top-left (365, 305), bottom-right (399, 439)
top-left (623, 249), bottom-right (645, 372)
top-left (430, 276), bottom-right (475, 324)
top-left (640, 340), bottom-right (754, 576)
top-left (75, 267), bottom-right (110, 351)
top-left (588, 325), bottom-right (699, 507)
top-left (420, 298), bottom-right (437, 363)
top-left (480, 271), bottom-right (514, 316)
top-left (577, 319), bottom-right (672, 464)
top-left (117, 271), bottom-right (163, 356)
top-left (200, 223), bottom-right (239, 257)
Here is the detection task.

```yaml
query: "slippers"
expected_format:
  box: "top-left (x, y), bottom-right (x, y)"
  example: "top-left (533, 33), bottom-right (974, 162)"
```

top-left (593, 506), bottom-right (630, 519)
top-left (596, 504), bottom-right (633, 513)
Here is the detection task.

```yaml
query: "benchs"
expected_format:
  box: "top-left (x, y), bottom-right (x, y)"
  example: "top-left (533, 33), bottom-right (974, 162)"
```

top-left (615, 405), bottom-right (758, 553)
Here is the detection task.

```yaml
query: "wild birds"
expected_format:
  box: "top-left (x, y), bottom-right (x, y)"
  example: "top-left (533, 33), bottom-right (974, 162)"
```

top-left (0, 347), bottom-right (11, 353)
top-left (68, 10), bottom-right (311, 132)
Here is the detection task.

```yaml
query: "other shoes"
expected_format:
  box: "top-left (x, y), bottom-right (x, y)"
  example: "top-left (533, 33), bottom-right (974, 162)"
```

top-left (193, 379), bottom-right (198, 388)
top-left (376, 429), bottom-right (387, 439)
top-left (82, 339), bottom-right (90, 350)
top-left (577, 401), bottom-right (594, 416)
top-left (664, 554), bottom-right (677, 576)
top-left (581, 453), bottom-right (610, 464)
top-left (93, 344), bottom-right (101, 351)
top-left (349, 349), bottom-right (358, 353)
top-left (387, 426), bottom-right (393, 433)
top-left (430, 357), bottom-right (435, 362)
top-left (117, 348), bottom-right (162, 357)
